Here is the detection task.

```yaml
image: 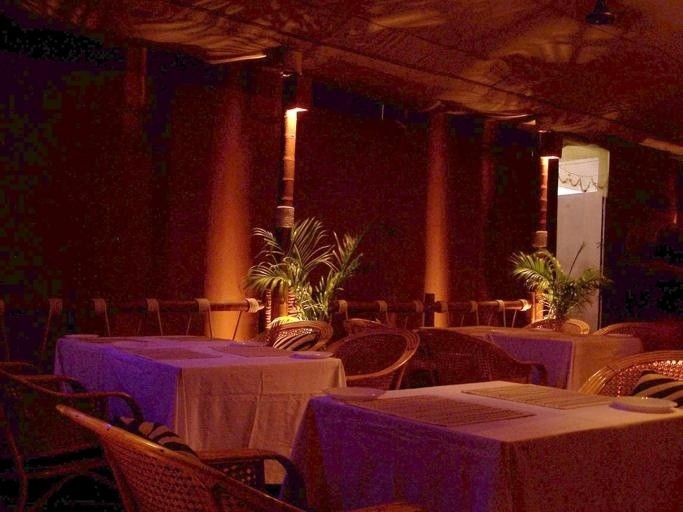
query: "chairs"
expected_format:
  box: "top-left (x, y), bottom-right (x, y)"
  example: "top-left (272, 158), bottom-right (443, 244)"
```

top-left (2, 357), bottom-right (93, 395)
top-left (344, 319), bottom-right (405, 336)
top-left (575, 348), bottom-right (682, 411)
top-left (241, 319), bottom-right (332, 356)
top-left (590, 319), bottom-right (680, 354)
top-left (521, 318), bottom-right (590, 338)
top-left (0, 370), bottom-right (150, 511)
top-left (418, 324), bottom-right (542, 388)
top-left (53, 404), bottom-right (428, 512)
top-left (320, 322), bottom-right (420, 391)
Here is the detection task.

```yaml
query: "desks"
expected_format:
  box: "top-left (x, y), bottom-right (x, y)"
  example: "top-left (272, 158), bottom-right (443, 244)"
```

top-left (307, 375), bottom-right (683, 512)
top-left (411, 319), bottom-right (646, 396)
top-left (59, 328), bottom-right (350, 480)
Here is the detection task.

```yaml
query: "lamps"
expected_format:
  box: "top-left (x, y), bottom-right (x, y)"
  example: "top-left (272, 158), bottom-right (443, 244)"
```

top-left (540, 132), bottom-right (562, 160)
top-left (283, 78), bottom-right (312, 111)
